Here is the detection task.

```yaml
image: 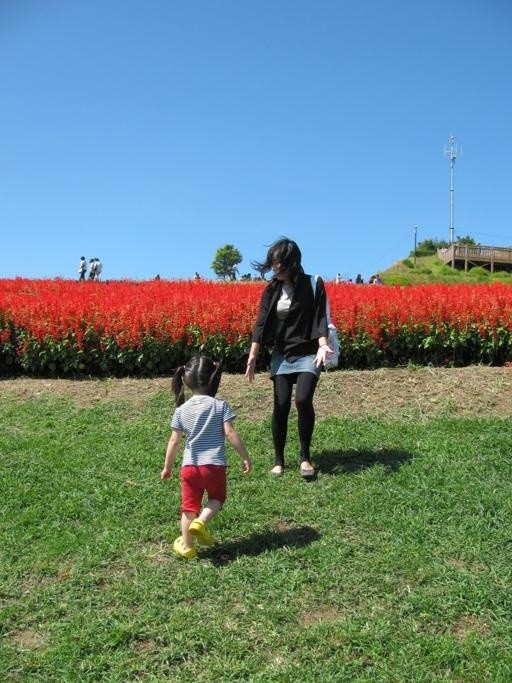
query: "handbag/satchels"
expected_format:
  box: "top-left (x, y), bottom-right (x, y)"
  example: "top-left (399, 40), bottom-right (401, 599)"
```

top-left (323, 323), bottom-right (339, 369)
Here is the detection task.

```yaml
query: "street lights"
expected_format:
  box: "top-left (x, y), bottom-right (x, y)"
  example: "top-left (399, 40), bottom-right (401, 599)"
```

top-left (413, 223), bottom-right (419, 264)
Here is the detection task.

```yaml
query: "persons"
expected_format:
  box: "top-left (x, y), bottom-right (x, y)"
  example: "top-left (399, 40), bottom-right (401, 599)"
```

top-left (348, 279), bottom-right (352, 284)
top-left (230, 264), bottom-right (265, 281)
top-left (194, 271), bottom-right (200, 280)
top-left (335, 272), bottom-right (341, 283)
top-left (79, 256), bottom-right (87, 282)
top-left (245, 236), bottom-right (334, 478)
top-left (88, 258), bottom-right (95, 280)
top-left (368, 275), bottom-right (376, 283)
top-left (92, 257), bottom-right (102, 281)
top-left (155, 274), bottom-right (160, 279)
top-left (160, 356), bottom-right (252, 557)
top-left (195, 275), bottom-right (201, 280)
top-left (356, 273), bottom-right (363, 283)
top-left (373, 273), bottom-right (383, 284)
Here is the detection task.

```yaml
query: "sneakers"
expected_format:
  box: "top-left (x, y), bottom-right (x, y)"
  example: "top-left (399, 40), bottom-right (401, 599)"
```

top-left (270, 465), bottom-right (283, 477)
top-left (189, 518), bottom-right (215, 546)
top-left (173, 536), bottom-right (197, 559)
top-left (301, 461), bottom-right (315, 476)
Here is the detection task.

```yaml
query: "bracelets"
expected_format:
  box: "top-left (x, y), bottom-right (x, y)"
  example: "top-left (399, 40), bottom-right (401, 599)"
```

top-left (318, 342), bottom-right (327, 347)
